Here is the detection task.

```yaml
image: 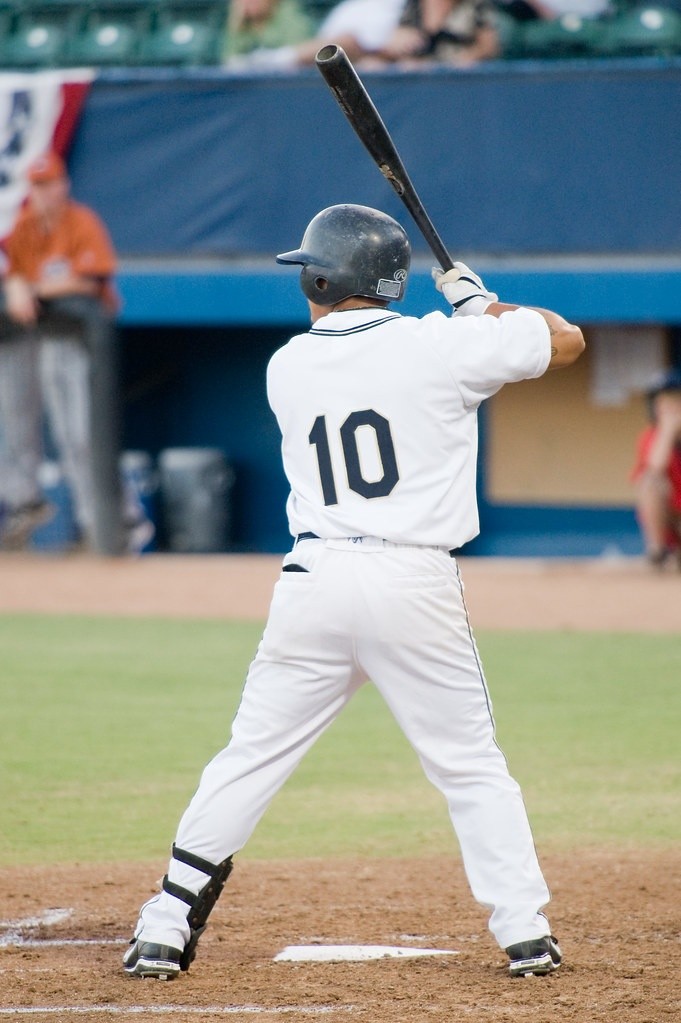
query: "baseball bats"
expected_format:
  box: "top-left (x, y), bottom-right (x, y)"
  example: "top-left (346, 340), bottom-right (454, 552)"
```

top-left (312, 41), bottom-right (457, 275)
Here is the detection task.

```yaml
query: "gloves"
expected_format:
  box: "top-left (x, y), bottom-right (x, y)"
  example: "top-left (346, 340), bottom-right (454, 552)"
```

top-left (432, 261), bottom-right (499, 317)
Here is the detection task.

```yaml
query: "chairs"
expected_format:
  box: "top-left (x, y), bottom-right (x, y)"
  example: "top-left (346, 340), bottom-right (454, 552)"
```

top-left (1, 0), bottom-right (681, 64)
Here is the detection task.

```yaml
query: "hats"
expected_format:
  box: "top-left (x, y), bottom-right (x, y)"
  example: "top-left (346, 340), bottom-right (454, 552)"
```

top-left (25, 150), bottom-right (65, 184)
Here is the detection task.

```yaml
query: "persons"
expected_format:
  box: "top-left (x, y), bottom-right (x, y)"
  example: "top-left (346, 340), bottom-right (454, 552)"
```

top-left (633, 372), bottom-right (681, 564)
top-left (122, 204), bottom-right (586, 975)
top-left (0, 151), bottom-right (119, 557)
top-left (218, 0), bottom-right (501, 73)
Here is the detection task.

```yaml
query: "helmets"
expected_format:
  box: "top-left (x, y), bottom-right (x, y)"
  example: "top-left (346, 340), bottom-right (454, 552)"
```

top-left (277, 202), bottom-right (413, 308)
top-left (642, 368), bottom-right (681, 426)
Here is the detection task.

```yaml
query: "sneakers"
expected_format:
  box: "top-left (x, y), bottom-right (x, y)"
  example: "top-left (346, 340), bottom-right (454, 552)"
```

top-left (122, 938), bottom-right (182, 980)
top-left (506, 935), bottom-right (562, 977)
